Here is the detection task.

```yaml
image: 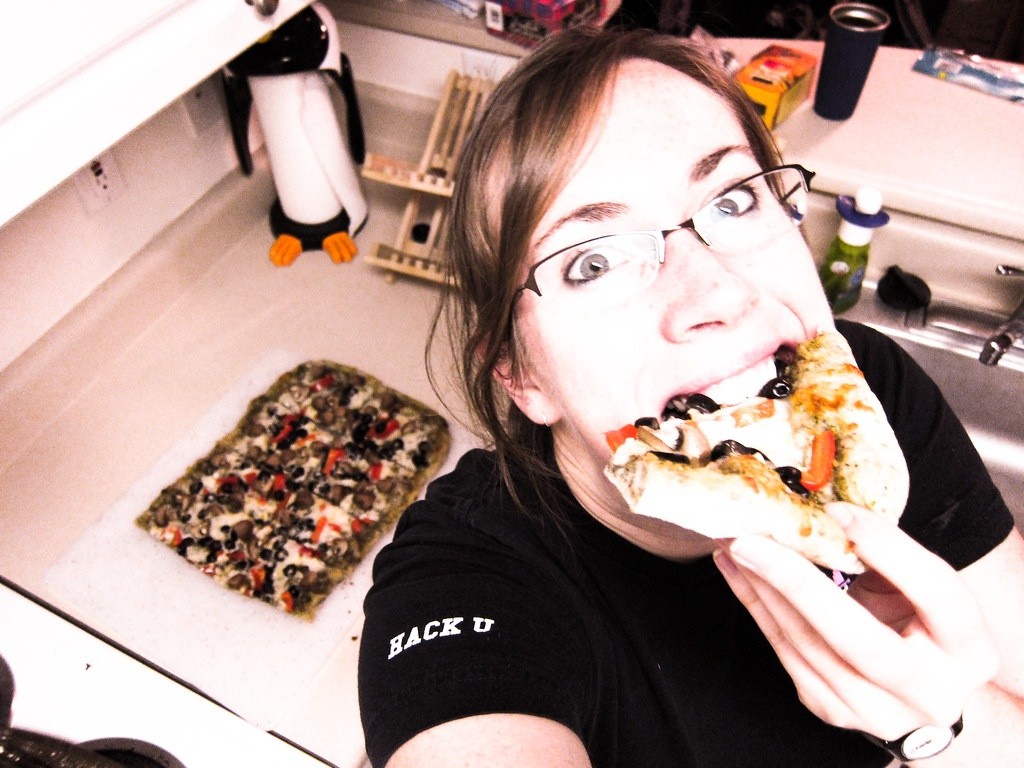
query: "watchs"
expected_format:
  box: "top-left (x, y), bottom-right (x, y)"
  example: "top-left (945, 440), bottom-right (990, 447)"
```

top-left (862, 710), bottom-right (968, 763)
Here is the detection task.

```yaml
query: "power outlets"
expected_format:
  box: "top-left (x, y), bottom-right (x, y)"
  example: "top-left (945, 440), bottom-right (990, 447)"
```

top-left (180, 77), bottom-right (226, 139)
top-left (76, 150), bottom-right (127, 217)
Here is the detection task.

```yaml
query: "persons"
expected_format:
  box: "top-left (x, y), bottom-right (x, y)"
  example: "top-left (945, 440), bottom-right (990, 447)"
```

top-left (348, 28), bottom-right (1024, 767)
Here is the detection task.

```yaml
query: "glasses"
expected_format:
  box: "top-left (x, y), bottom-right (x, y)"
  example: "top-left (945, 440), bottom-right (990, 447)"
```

top-left (510, 162), bottom-right (819, 307)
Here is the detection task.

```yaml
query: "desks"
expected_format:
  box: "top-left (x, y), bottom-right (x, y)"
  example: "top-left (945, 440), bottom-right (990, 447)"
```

top-left (678, 34), bottom-right (1022, 244)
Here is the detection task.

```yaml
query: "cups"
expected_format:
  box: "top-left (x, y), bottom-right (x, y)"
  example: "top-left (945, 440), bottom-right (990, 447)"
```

top-left (814, 2), bottom-right (892, 120)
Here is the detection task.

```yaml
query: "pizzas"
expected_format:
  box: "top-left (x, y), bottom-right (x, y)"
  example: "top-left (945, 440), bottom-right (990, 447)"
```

top-left (134, 357), bottom-right (448, 621)
top-left (605, 325), bottom-right (910, 578)
